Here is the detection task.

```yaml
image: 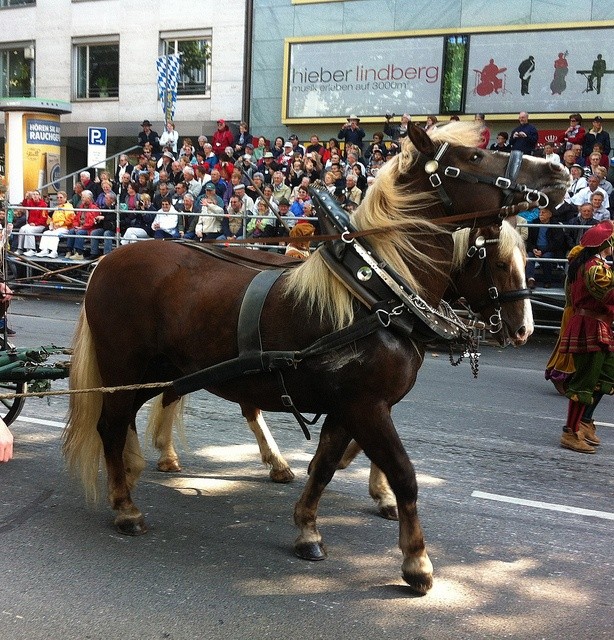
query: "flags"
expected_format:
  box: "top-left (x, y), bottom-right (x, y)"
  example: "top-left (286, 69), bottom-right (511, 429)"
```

top-left (155, 55), bottom-right (181, 132)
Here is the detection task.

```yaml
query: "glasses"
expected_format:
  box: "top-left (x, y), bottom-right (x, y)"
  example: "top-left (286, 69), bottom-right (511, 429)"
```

top-left (56, 197), bottom-right (62, 198)
top-left (569, 120), bottom-right (575, 122)
top-left (299, 192), bottom-right (304, 193)
top-left (144, 152), bottom-right (149, 153)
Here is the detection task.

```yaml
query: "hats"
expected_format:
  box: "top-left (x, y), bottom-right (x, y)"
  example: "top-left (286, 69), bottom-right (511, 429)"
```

top-left (263, 152), bottom-right (275, 158)
top-left (289, 135), bottom-right (297, 140)
top-left (216, 119), bottom-right (224, 124)
top-left (204, 182), bottom-right (215, 191)
top-left (284, 142), bottom-right (293, 148)
top-left (374, 148), bottom-right (382, 154)
top-left (568, 164), bottom-right (584, 177)
top-left (244, 143), bottom-right (253, 150)
top-left (140, 120), bottom-right (151, 126)
top-left (594, 116), bottom-right (602, 122)
top-left (347, 114), bottom-right (360, 122)
top-left (334, 186), bottom-right (346, 195)
top-left (276, 198), bottom-right (290, 206)
top-left (580, 219), bottom-right (613, 248)
top-left (233, 184), bottom-right (245, 192)
top-left (162, 151), bottom-right (174, 162)
top-left (289, 223), bottom-right (314, 246)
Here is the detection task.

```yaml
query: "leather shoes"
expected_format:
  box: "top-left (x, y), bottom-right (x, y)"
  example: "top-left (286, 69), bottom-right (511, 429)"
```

top-left (528, 280), bottom-right (535, 289)
top-left (543, 284), bottom-right (551, 288)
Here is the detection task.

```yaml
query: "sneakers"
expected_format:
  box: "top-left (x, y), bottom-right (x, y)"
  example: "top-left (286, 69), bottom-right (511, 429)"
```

top-left (13, 249), bottom-right (22, 255)
top-left (64, 252), bottom-right (71, 259)
top-left (69, 253), bottom-right (83, 260)
top-left (0, 327), bottom-right (16, 337)
top-left (35, 250), bottom-right (49, 257)
top-left (23, 250), bottom-right (36, 256)
top-left (47, 251), bottom-right (58, 259)
top-left (86, 254), bottom-right (98, 259)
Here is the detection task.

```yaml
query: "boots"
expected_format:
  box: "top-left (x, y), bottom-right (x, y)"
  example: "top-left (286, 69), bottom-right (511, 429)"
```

top-left (559, 426), bottom-right (595, 453)
top-left (580, 417), bottom-right (600, 445)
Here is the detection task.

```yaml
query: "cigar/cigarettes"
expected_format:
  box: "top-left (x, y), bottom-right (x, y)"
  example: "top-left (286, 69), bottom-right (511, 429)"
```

top-left (37, 154), bottom-right (46, 195)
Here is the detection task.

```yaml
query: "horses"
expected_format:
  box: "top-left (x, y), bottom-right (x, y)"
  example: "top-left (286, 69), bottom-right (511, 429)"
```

top-left (143, 219), bottom-right (535, 521)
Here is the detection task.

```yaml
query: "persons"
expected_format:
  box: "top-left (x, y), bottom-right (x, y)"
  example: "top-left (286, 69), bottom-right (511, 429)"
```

top-left (76, 114), bottom-right (419, 259)
top-left (0, 210), bottom-right (8, 225)
top-left (473, 112), bottom-right (491, 151)
top-left (13, 188), bottom-right (45, 257)
top-left (594, 165), bottom-right (613, 193)
top-left (38, 191), bottom-right (74, 259)
top-left (579, 175), bottom-right (609, 206)
top-left (0, 413), bottom-right (16, 467)
top-left (510, 112), bottom-right (539, 156)
top-left (526, 206), bottom-right (566, 288)
top-left (583, 116), bottom-right (612, 159)
top-left (558, 114), bottom-right (585, 144)
top-left (423, 112), bottom-right (439, 132)
top-left (0, 318), bottom-right (17, 336)
top-left (590, 144), bottom-right (610, 170)
top-left (0, 225), bottom-right (10, 260)
top-left (449, 115), bottom-right (459, 121)
top-left (490, 131), bottom-right (509, 151)
top-left (590, 191), bottom-right (611, 223)
top-left (584, 152), bottom-right (602, 181)
top-left (0, 278), bottom-right (13, 317)
top-left (542, 143), bottom-right (587, 195)
top-left (569, 201), bottom-right (598, 247)
top-left (543, 219), bottom-right (614, 454)
